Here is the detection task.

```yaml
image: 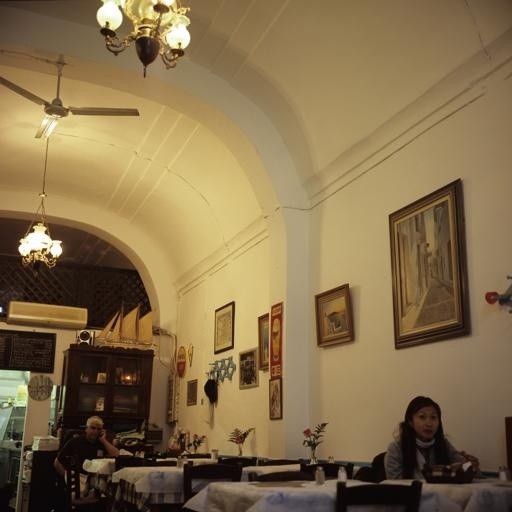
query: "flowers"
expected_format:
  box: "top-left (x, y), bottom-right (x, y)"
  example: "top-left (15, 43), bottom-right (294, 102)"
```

top-left (227, 427), bottom-right (256, 446)
top-left (303, 422), bottom-right (329, 451)
top-left (193, 434), bottom-right (206, 450)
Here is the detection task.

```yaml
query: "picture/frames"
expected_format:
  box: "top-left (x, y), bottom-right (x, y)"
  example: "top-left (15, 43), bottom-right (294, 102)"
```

top-left (258, 313), bottom-right (269, 369)
top-left (214, 301), bottom-right (233, 354)
top-left (315, 284), bottom-right (354, 347)
top-left (268, 378), bottom-right (282, 419)
top-left (239, 346), bottom-right (259, 391)
top-left (187, 380), bottom-right (197, 405)
top-left (389, 178), bottom-right (471, 349)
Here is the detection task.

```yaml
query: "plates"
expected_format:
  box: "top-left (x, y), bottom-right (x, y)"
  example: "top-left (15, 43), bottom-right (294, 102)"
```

top-left (420, 467), bottom-right (476, 483)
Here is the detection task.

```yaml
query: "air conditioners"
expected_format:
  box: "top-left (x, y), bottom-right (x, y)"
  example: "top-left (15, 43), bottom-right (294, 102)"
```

top-left (7, 302), bottom-right (88, 329)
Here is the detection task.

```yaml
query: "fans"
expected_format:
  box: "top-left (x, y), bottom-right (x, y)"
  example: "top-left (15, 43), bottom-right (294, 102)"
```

top-left (0, 54), bottom-right (140, 141)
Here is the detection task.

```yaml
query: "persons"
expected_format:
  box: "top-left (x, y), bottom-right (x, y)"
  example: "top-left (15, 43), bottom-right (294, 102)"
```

top-left (55, 416), bottom-right (120, 508)
top-left (384, 396), bottom-right (475, 481)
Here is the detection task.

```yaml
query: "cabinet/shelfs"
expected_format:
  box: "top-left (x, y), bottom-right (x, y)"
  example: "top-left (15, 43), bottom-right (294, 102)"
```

top-left (58, 343), bottom-right (154, 440)
top-left (21, 444), bottom-right (59, 512)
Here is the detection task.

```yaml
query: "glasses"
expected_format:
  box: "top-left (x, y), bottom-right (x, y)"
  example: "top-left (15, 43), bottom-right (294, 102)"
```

top-left (90, 425), bottom-right (102, 431)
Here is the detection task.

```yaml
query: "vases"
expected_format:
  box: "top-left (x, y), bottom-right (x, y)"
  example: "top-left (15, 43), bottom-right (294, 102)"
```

top-left (239, 444), bottom-right (243, 456)
top-left (310, 450), bottom-right (317, 465)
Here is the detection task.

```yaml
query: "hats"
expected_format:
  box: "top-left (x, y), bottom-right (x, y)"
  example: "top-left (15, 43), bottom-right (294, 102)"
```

top-left (204, 379), bottom-right (218, 405)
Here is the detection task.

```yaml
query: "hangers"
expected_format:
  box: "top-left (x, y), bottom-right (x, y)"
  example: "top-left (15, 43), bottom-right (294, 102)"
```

top-left (205, 356), bottom-right (236, 382)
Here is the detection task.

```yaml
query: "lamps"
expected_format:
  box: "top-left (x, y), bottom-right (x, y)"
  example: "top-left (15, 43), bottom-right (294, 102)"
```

top-left (18, 136), bottom-right (63, 270)
top-left (96, 0), bottom-right (191, 77)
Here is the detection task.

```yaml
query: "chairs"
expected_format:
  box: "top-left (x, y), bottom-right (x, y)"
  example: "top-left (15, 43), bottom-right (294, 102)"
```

top-left (337, 481), bottom-right (423, 512)
top-left (183, 464), bottom-right (242, 505)
top-left (67, 469), bottom-right (102, 508)
top-left (298, 459), bottom-right (354, 480)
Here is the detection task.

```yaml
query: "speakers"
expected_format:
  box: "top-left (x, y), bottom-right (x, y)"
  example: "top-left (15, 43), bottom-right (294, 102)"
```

top-left (76, 330), bottom-right (95, 347)
